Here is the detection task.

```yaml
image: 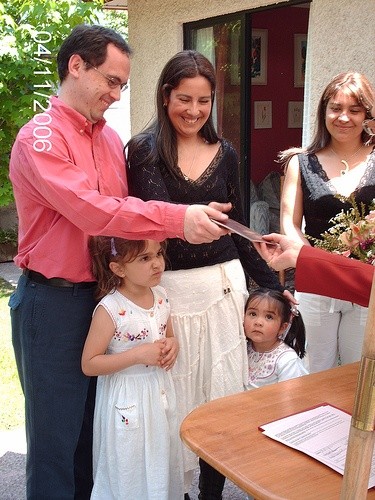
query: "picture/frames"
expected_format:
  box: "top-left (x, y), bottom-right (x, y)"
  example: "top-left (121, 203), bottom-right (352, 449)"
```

top-left (230, 28), bottom-right (308, 129)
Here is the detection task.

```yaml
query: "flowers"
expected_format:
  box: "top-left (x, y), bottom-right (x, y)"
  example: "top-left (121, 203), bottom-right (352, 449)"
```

top-left (302, 193), bottom-right (375, 266)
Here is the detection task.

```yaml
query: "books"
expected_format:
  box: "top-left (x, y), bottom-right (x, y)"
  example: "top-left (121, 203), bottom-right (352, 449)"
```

top-left (210, 217), bottom-right (276, 248)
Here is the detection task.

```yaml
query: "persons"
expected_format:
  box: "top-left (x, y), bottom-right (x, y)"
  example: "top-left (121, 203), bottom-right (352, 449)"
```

top-left (273, 68), bottom-right (375, 379)
top-left (80, 233), bottom-right (192, 500)
top-left (6, 25), bottom-right (234, 500)
top-left (241, 286), bottom-right (311, 391)
top-left (251, 232), bottom-right (375, 309)
top-left (121, 48), bottom-right (299, 499)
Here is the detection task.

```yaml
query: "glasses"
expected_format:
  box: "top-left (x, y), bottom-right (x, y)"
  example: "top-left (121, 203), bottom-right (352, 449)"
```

top-left (84, 60), bottom-right (128, 92)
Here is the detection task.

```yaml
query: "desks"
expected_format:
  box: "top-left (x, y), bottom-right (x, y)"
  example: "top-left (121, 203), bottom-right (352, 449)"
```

top-left (179, 360), bottom-right (375, 500)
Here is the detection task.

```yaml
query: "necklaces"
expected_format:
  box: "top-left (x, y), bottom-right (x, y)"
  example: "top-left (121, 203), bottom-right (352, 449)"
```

top-left (175, 139), bottom-right (202, 181)
top-left (328, 143), bottom-right (366, 175)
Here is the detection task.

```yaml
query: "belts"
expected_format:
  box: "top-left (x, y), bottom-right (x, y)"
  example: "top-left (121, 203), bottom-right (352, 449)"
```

top-left (23, 268), bottom-right (99, 289)
